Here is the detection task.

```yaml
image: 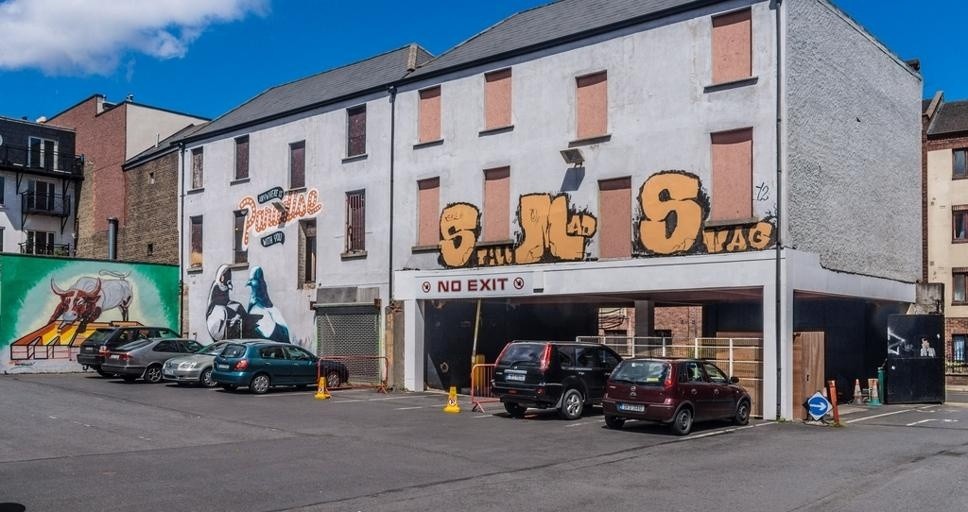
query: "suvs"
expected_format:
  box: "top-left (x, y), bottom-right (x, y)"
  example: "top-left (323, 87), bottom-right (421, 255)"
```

top-left (490, 338), bottom-right (623, 420)
top-left (76, 326), bottom-right (182, 376)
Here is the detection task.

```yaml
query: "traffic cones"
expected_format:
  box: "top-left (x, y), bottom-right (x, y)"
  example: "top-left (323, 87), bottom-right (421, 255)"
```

top-left (852, 379), bottom-right (882, 406)
top-left (443, 385), bottom-right (461, 413)
top-left (314, 376), bottom-right (331, 400)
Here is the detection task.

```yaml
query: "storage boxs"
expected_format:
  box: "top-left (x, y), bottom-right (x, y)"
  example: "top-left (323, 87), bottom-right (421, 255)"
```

top-left (714, 331), bottom-right (763, 417)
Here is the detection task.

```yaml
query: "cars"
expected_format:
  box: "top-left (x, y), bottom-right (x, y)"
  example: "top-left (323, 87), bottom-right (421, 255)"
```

top-left (161, 338), bottom-right (280, 388)
top-left (602, 354), bottom-right (752, 435)
top-left (99, 337), bottom-right (207, 385)
top-left (210, 341), bottom-right (349, 395)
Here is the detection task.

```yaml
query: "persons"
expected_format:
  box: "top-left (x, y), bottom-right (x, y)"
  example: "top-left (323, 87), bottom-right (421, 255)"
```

top-left (920, 337), bottom-right (936, 357)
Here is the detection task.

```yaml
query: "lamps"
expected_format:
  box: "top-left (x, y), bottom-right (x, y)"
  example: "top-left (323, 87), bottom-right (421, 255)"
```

top-left (272, 201), bottom-right (287, 213)
top-left (559, 148), bottom-right (584, 166)
